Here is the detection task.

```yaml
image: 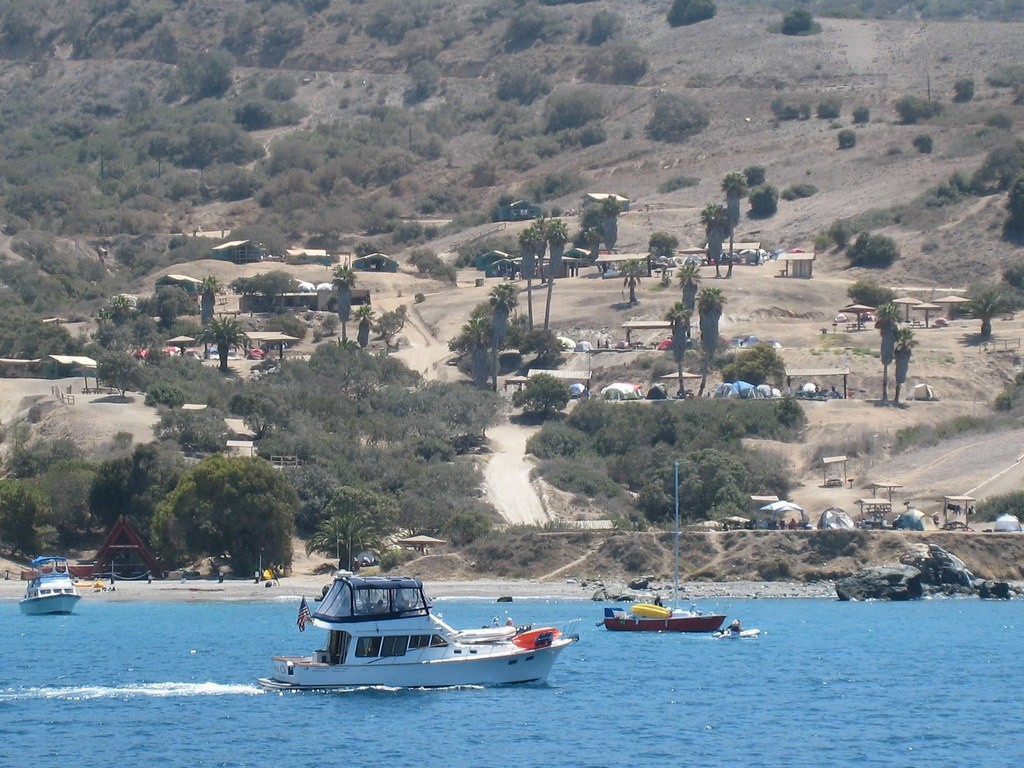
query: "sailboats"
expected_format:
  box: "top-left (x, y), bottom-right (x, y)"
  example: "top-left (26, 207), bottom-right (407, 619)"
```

top-left (589, 461), bottom-right (729, 632)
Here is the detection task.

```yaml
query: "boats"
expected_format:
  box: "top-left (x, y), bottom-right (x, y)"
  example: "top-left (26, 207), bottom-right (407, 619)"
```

top-left (254, 569), bottom-right (583, 693)
top-left (19, 553), bottom-right (82, 614)
top-left (712, 627), bottom-right (761, 640)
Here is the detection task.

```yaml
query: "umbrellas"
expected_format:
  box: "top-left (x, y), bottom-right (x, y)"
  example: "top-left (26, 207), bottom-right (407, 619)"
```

top-left (168, 335), bottom-right (195, 355)
top-left (841, 294), bottom-right (971, 331)
top-left (399, 534), bottom-right (446, 556)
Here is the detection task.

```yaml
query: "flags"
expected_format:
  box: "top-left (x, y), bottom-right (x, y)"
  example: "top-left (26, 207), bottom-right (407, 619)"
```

top-left (296, 598), bottom-right (310, 632)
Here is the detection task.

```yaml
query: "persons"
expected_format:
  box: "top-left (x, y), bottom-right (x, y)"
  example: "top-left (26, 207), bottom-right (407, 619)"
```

top-left (724, 518), bottom-right (806, 529)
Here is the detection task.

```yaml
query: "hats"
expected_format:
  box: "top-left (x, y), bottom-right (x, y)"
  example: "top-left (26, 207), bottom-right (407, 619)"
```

top-left (507, 617), bottom-right (513, 621)
top-left (492, 616), bottom-right (499, 622)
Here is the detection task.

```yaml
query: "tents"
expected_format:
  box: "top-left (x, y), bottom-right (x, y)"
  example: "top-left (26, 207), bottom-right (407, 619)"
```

top-left (569, 383), bottom-right (586, 399)
top-left (578, 192), bottom-right (630, 213)
top-left (760, 501), bottom-right (805, 527)
top-left (475, 250), bottom-right (515, 278)
top-left (212, 240), bottom-right (262, 264)
top-left (497, 200), bottom-right (541, 221)
top-left (712, 381), bottom-right (783, 399)
top-left (659, 339), bottom-right (673, 349)
top-left (617, 340), bottom-right (628, 348)
top-left (835, 311), bottom-right (876, 322)
top-left (801, 383), bottom-right (820, 393)
top-left (906, 383), bottom-right (941, 400)
top-left (576, 342), bottom-right (592, 351)
top-left (155, 274), bottom-right (201, 295)
top-left (817, 506), bottom-right (855, 529)
top-left (729, 336), bottom-right (783, 350)
top-left (563, 247), bottom-right (590, 264)
top-left (351, 253), bottom-right (400, 274)
top-left (134, 345), bottom-right (265, 361)
top-left (645, 384), bottom-right (669, 399)
top-left (655, 248), bottom-right (804, 268)
top-left (602, 383), bottom-right (640, 400)
top-left (995, 516), bottom-right (1022, 531)
top-left (896, 509), bottom-right (939, 531)
top-left (299, 281), bottom-right (333, 292)
